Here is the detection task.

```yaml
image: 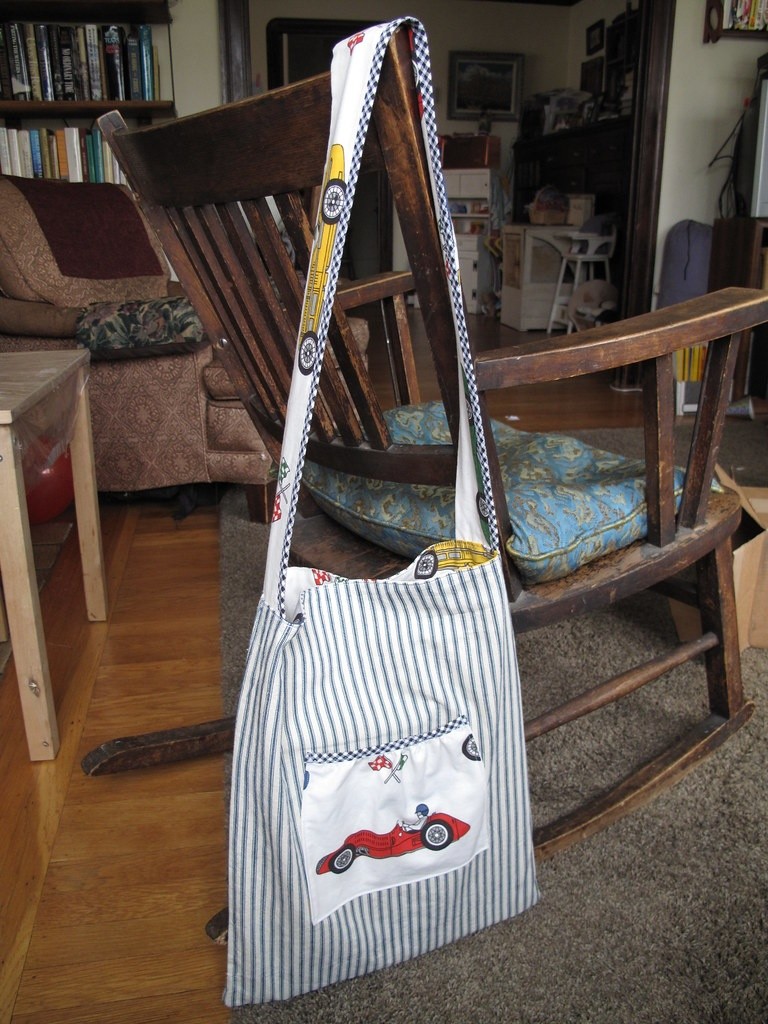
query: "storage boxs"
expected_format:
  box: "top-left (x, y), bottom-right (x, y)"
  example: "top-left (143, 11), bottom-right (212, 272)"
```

top-left (668, 464), bottom-right (768, 655)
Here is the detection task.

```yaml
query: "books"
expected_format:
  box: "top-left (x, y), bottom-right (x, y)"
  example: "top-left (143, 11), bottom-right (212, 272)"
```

top-left (0, 21), bottom-right (173, 101)
top-left (0, 126), bottom-right (132, 190)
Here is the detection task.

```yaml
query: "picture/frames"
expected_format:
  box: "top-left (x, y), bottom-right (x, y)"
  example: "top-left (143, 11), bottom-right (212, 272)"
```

top-left (580, 56), bottom-right (604, 95)
top-left (585, 18), bottom-right (605, 56)
top-left (446, 50), bottom-right (526, 123)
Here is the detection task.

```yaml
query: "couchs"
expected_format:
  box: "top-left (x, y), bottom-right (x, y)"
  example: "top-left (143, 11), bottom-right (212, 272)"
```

top-left (0, 174), bottom-right (280, 525)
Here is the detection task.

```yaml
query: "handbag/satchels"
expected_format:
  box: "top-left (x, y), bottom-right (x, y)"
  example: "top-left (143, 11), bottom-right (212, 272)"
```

top-left (223, 541), bottom-right (540, 1010)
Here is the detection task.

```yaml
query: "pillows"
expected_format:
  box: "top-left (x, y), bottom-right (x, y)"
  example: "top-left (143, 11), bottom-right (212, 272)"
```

top-left (75, 295), bottom-right (210, 362)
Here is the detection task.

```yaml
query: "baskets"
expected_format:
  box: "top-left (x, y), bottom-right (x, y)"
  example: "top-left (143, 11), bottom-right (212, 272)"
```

top-left (528, 186), bottom-right (567, 225)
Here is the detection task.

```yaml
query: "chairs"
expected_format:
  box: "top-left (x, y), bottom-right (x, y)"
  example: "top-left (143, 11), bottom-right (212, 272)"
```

top-left (81, 25), bottom-right (768, 949)
top-left (547, 224), bottom-right (617, 335)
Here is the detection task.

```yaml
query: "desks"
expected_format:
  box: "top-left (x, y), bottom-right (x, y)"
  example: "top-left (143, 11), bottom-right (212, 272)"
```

top-left (0, 349), bottom-right (108, 763)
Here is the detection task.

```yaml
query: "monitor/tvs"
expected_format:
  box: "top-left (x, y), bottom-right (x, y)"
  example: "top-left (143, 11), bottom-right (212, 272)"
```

top-left (441, 132), bottom-right (501, 169)
top-left (728, 70), bottom-right (768, 218)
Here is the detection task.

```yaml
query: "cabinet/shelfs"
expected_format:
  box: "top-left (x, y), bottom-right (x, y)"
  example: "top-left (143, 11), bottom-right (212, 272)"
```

top-left (513, 113), bottom-right (635, 322)
top-left (708, 217), bottom-right (768, 414)
top-left (0, 0), bottom-right (178, 280)
top-left (414, 168), bottom-right (502, 315)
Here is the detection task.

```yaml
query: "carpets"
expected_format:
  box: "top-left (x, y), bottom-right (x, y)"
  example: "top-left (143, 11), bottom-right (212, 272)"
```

top-left (216, 418), bottom-right (768, 1024)
